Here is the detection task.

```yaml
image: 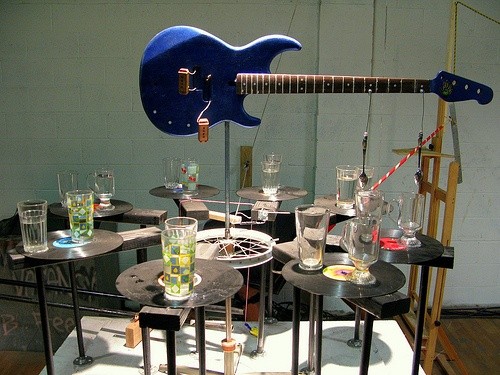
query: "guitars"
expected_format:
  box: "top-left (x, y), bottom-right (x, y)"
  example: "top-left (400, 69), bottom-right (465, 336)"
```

top-left (138, 25), bottom-right (494, 138)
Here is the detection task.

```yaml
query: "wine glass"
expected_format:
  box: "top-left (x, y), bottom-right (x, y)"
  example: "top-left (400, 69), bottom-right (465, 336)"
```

top-left (343, 216), bottom-right (381, 286)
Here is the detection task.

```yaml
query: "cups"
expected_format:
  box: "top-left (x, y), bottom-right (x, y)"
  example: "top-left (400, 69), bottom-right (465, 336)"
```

top-left (86, 170), bottom-right (115, 213)
top-left (165, 217), bottom-right (199, 231)
top-left (57, 170), bottom-right (78, 208)
top-left (161, 228), bottom-right (197, 301)
top-left (264, 152), bottom-right (282, 162)
top-left (162, 158), bottom-right (183, 190)
top-left (294, 203), bottom-right (330, 271)
top-left (17, 199), bottom-right (47, 254)
top-left (354, 165), bottom-right (375, 207)
top-left (259, 160), bottom-right (281, 196)
top-left (335, 164), bottom-right (359, 209)
top-left (387, 192), bottom-right (426, 248)
top-left (355, 189), bottom-right (385, 247)
top-left (66, 189), bottom-right (94, 243)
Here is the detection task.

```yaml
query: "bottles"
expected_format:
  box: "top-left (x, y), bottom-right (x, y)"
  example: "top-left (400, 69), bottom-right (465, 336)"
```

top-left (187, 160), bottom-right (200, 192)
